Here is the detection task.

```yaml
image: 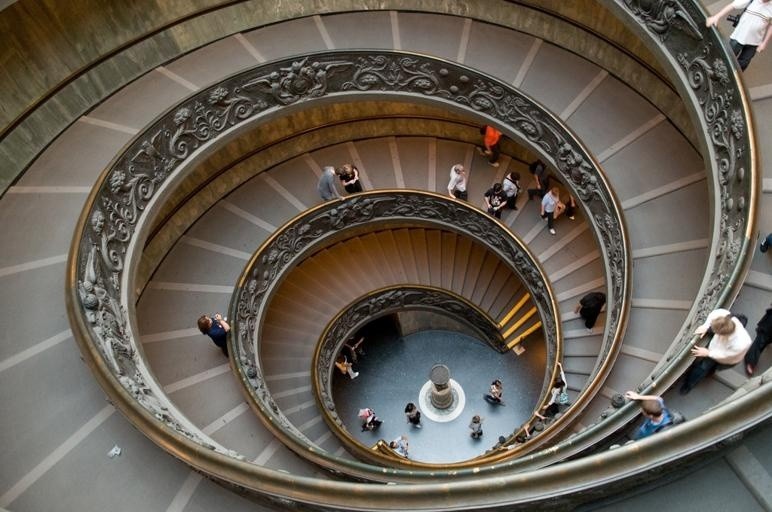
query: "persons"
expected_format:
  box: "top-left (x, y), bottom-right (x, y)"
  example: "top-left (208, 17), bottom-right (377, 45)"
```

top-left (389, 434), bottom-right (411, 459)
top-left (195, 311), bottom-right (231, 361)
top-left (707, 0), bottom-right (772, 73)
top-left (470, 414), bottom-right (484, 441)
top-left (335, 354), bottom-right (361, 382)
top-left (355, 406), bottom-right (382, 433)
top-left (317, 164), bottom-right (346, 202)
top-left (484, 376), bottom-right (507, 408)
top-left (500, 363), bottom-right (568, 454)
top-left (625, 231), bottom-right (772, 450)
top-left (445, 119), bottom-right (580, 253)
top-left (339, 161), bottom-right (366, 193)
top-left (403, 400), bottom-right (423, 431)
top-left (574, 290), bottom-right (607, 333)
top-left (344, 334), bottom-right (366, 361)
